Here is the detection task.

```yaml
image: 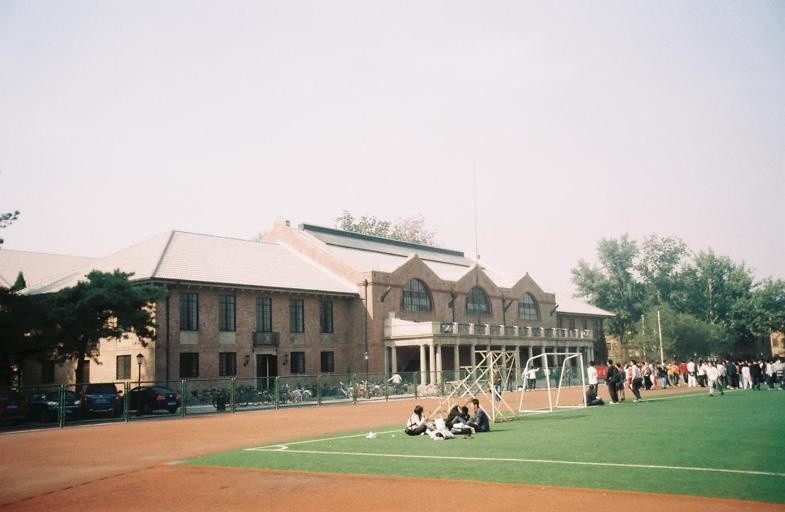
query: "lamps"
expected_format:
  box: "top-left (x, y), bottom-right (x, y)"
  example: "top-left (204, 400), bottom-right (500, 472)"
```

top-left (243, 352), bottom-right (250, 366)
top-left (282, 353), bottom-right (289, 365)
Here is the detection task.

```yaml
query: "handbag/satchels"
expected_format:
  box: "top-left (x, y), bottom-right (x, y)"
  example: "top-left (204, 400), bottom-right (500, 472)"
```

top-left (405, 427), bottom-right (414, 436)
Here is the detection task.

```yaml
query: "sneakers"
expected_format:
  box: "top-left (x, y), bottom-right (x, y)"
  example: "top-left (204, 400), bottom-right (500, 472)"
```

top-left (709, 393), bottom-right (714, 397)
top-left (721, 392), bottom-right (724, 396)
top-left (641, 382), bottom-right (782, 391)
top-left (609, 400), bottom-right (619, 405)
top-left (633, 396), bottom-right (642, 402)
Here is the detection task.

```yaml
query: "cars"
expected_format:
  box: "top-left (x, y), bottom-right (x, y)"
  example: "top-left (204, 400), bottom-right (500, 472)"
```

top-left (0, 383), bottom-right (181, 425)
top-left (186, 379), bottom-right (413, 408)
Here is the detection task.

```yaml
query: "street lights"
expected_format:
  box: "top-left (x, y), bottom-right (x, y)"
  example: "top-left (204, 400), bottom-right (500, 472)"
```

top-left (136, 352), bottom-right (143, 387)
top-left (363, 351), bottom-right (370, 399)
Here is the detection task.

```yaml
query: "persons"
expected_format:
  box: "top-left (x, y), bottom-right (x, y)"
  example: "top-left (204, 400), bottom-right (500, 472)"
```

top-left (388, 373), bottom-right (402, 384)
top-left (466, 399), bottom-right (490, 433)
top-left (447, 405), bottom-right (470, 429)
top-left (490, 363), bottom-right (571, 403)
top-left (583, 354), bottom-right (785, 406)
top-left (405, 406), bottom-right (427, 436)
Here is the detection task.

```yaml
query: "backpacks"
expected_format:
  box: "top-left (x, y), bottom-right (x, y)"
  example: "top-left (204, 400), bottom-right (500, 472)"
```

top-left (612, 369), bottom-right (621, 383)
